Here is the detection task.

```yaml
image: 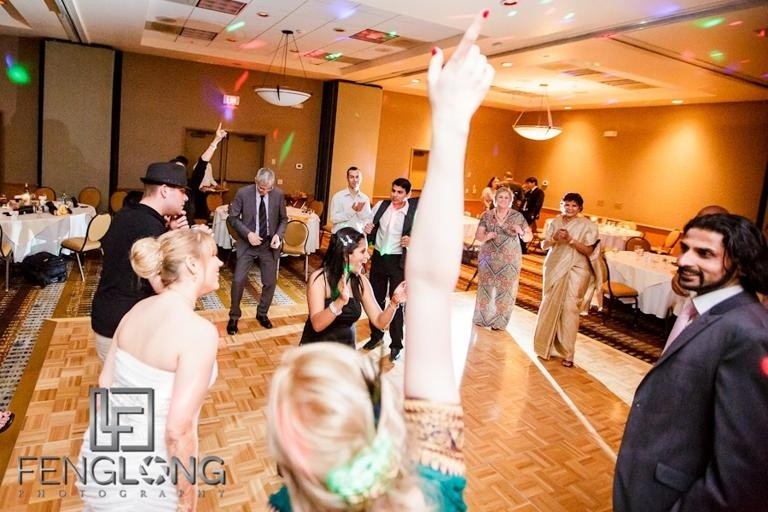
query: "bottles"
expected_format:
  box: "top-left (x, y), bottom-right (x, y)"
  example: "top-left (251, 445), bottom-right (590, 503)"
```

top-left (24, 181), bottom-right (30, 197)
top-left (61, 192), bottom-right (67, 203)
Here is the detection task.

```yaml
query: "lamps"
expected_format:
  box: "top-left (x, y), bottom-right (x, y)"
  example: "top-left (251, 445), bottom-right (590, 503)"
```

top-left (252, 26), bottom-right (312, 109)
top-left (512, 83), bottom-right (565, 142)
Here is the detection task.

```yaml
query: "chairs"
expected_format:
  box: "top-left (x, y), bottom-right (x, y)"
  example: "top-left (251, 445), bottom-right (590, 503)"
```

top-left (598, 247), bottom-right (640, 318)
top-left (648, 228), bottom-right (682, 255)
top-left (206, 192), bottom-right (223, 213)
top-left (77, 184), bottom-right (103, 217)
top-left (625, 235), bottom-right (652, 253)
top-left (617, 219), bottom-right (639, 230)
top-left (58, 213), bottom-right (114, 279)
top-left (277, 219), bottom-right (310, 282)
top-left (309, 199), bottom-right (324, 217)
top-left (34, 185), bottom-right (56, 204)
top-left (111, 189), bottom-right (128, 212)
top-left (1, 222), bottom-right (15, 293)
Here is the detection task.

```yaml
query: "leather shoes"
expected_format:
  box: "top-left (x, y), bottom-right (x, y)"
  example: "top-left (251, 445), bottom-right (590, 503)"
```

top-left (254, 314), bottom-right (273, 330)
top-left (226, 316), bottom-right (239, 336)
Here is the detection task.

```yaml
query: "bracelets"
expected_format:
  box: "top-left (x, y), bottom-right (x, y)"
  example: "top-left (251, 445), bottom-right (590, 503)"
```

top-left (569, 238), bottom-right (573, 245)
top-left (329, 301), bottom-right (342, 316)
top-left (389, 302), bottom-right (401, 312)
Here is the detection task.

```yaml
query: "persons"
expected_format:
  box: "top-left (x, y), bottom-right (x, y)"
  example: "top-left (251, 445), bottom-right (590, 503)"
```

top-left (473, 186), bottom-right (533, 331)
top-left (480, 176), bottom-right (501, 211)
top-left (534, 193), bottom-right (603, 367)
top-left (227, 168), bottom-right (289, 334)
top-left (518, 177), bottom-right (544, 254)
top-left (612, 213), bottom-right (768, 511)
top-left (330, 167), bottom-right (374, 251)
top-left (362, 178), bottom-right (416, 363)
top-left (298, 227), bottom-right (407, 350)
top-left (75, 223), bottom-right (224, 512)
top-left (168, 122), bottom-right (228, 229)
top-left (92, 162), bottom-right (192, 364)
top-left (490, 172), bottom-right (524, 210)
top-left (671, 206), bottom-right (730, 330)
top-left (264, 7), bottom-right (496, 512)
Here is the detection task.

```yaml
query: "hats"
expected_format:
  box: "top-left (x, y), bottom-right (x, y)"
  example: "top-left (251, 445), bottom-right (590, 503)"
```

top-left (138, 161), bottom-right (193, 192)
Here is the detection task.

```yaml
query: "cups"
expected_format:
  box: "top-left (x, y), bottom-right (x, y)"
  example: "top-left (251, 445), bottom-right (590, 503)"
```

top-left (12, 211), bottom-right (18, 221)
top-left (634, 245), bottom-right (643, 261)
top-left (36, 209), bottom-right (42, 218)
top-left (612, 246), bottom-right (619, 258)
top-left (560, 223), bottom-right (566, 231)
top-left (653, 255), bottom-right (659, 267)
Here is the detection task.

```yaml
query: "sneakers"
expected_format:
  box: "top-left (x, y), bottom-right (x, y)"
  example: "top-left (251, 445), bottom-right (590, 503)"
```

top-left (361, 338), bottom-right (384, 352)
top-left (389, 348), bottom-right (400, 359)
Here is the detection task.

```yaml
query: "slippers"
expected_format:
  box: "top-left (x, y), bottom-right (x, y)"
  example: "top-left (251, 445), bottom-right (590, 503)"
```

top-left (561, 359), bottom-right (574, 371)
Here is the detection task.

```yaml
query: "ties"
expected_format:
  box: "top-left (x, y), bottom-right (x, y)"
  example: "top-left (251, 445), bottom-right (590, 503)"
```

top-left (259, 195), bottom-right (269, 244)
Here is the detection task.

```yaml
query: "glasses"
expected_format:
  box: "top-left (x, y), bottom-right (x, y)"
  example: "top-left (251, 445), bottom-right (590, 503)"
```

top-left (256, 186), bottom-right (272, 191)
